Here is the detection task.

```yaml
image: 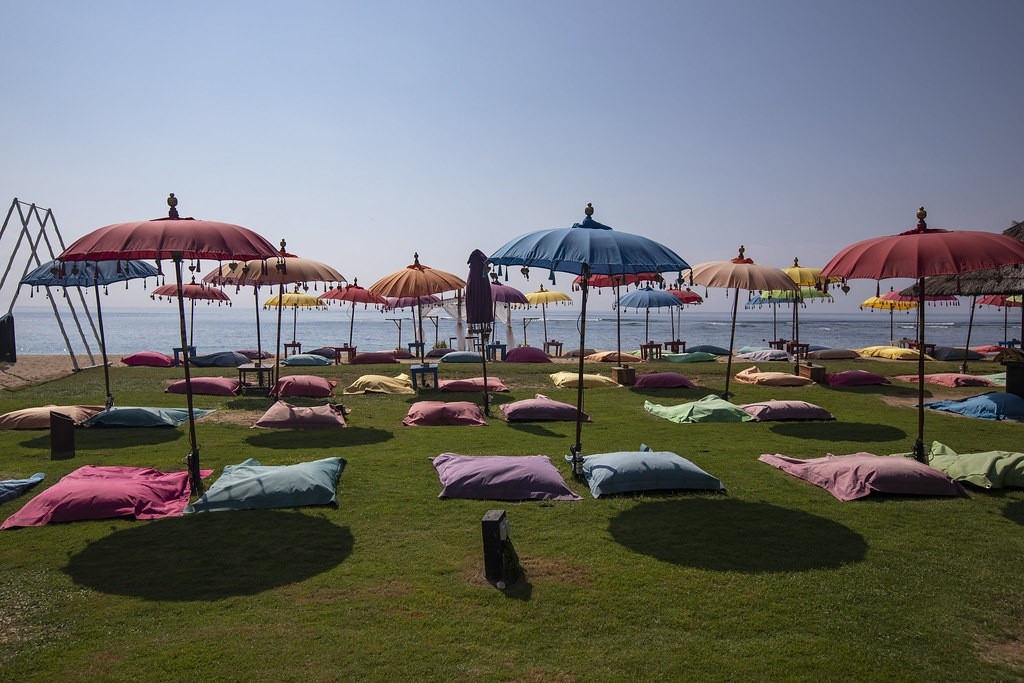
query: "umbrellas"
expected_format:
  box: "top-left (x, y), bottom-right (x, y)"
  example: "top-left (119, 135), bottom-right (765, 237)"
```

top-left (20, 194), bottom-right (1024, 498)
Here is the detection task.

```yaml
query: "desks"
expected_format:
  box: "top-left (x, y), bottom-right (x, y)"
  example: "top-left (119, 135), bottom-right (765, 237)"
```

top-left (639, 343), bottom-right (662, 360)
top-left (410, 364), bottom-right (439, 391)
top-left (664, 342), bottom-right (686, 354)
top-left (408, 342), bottom-right (425, 357)
top-left (611, 366), bottom-right (636, 384)
top-left (543, 342), bottom-right (563, 356)
top-left (237, 363), bottom-right (275, 396)
top-left (172, 347), bottom-right (197, 366)
top-left (889, 340), bottom-right (918, 348)
top-left (768, 340), bottom-right (798, 353)
top-left (786, 342), bottom-right (810, 359)
top-left (798, 363), bottom-right (826, 381)
top-left (908, 342), bottom-right (936, 354)
top-left (486, 344), bottom-right (507, 361)
top-left (333, 345), bottom-right (357, 365)
top-left (1000, 360), bottom-right (1024, 399)
top-left (284, 343), bottom-right (301, 358)
top-left (998, 340), bottom-right (1021, 349)
